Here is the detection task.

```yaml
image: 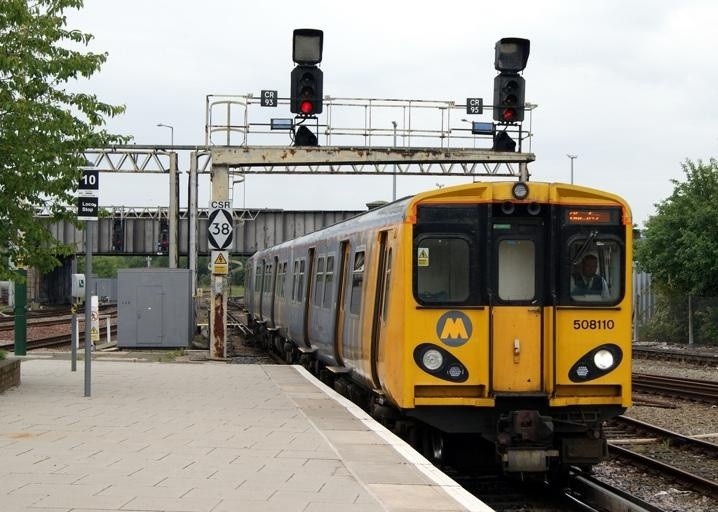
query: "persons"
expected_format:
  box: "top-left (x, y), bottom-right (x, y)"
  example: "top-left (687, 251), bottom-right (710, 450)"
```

top-left (570, 254), bottom-right (611, 303)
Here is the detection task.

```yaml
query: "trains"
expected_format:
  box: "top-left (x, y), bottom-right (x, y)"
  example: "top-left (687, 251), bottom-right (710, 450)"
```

top-left (243, 180), bottom-right (636, 482)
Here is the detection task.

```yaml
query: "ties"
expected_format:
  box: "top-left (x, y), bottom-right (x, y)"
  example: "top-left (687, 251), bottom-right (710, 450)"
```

top-left (585, 278), bottom-right (592, 288)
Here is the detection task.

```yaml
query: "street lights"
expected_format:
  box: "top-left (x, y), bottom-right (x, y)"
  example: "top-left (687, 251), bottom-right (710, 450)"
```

top-left (157, 123), bottom-right (174, 155)
top-left (567, 154), bottom-right (579, 184)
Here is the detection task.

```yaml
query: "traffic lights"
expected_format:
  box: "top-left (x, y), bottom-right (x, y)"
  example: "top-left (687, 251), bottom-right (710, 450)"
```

top-left (493, 73), bottom-right (525, 124)
top-left (160, 233), bottom-right (168, 251)
top-left (495, 37), bottom-right (532, 72)
top-left (290, 66), bottom-right (322, 115)
top-left (113, 229), bottom-right (123, 247)
top-left (291, 28), bottom-right (325, 64)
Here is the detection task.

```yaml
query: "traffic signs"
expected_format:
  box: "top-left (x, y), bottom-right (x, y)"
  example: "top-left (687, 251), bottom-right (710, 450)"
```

top-left (207, 210), bottom-right (237, 250)
